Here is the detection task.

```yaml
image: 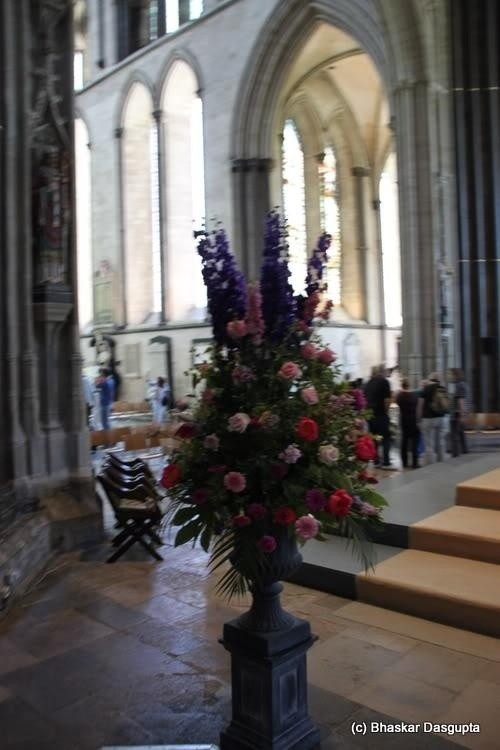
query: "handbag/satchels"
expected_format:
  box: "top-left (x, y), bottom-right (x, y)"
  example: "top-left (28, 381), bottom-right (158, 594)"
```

top-left (431, 393), bottom-right (447, 411)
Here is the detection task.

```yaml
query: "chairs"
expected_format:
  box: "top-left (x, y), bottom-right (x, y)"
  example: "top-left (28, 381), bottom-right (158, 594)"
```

top-left (95, 451), bottom-right (164, 565)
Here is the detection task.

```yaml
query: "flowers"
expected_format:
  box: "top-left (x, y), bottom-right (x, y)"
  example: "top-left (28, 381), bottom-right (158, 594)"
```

top-left (157, 203), bottom-right (395, 608)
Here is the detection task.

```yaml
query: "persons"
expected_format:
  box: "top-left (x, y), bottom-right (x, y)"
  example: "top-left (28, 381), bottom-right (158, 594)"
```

top-left (83, 367), bottom-right (115, 431)
top-left (145, 375), bottom-right (171, 430)
top-left (352, 363), bottom-right (473, 468)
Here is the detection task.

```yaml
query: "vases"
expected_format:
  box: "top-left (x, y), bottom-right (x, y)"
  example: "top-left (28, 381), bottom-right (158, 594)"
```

top-left (226, 515), bottom-right (304, 633)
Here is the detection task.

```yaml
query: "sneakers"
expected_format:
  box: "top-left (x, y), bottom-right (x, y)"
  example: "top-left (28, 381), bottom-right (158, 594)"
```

top-left (375, 462), bottom-right (399, 470)
